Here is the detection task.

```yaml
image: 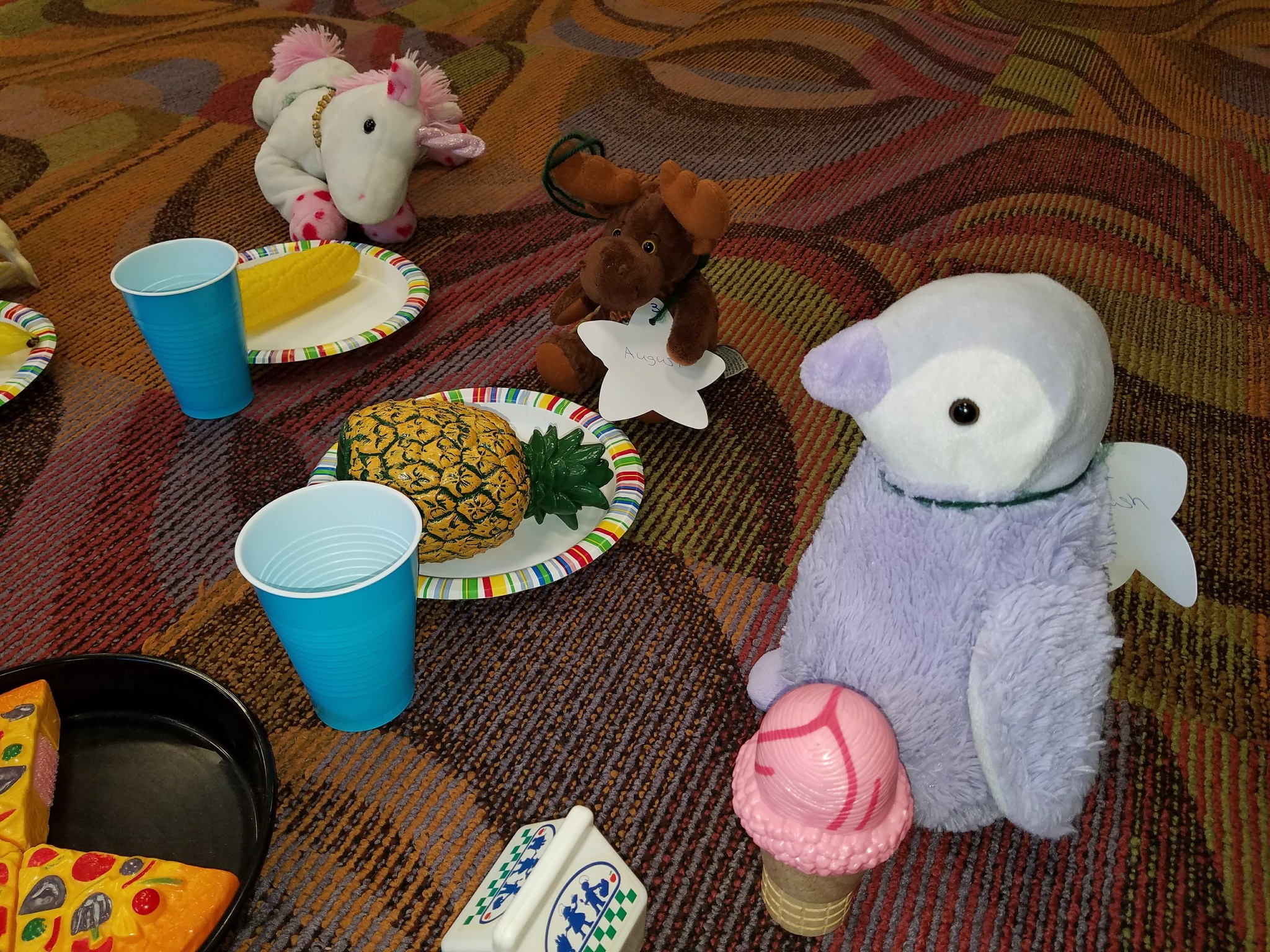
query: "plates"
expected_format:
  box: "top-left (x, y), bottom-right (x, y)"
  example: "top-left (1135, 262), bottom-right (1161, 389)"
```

top-left (235, 241), bottom-right (431, 361)
top-left (306, 388), bottom-right (647, 600)
top-left (0, 301), bottom-right (57, 407)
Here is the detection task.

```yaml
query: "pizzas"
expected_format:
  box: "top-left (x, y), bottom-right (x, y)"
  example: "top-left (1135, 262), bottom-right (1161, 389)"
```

top-left (0, 676), bottom-right (238, 952)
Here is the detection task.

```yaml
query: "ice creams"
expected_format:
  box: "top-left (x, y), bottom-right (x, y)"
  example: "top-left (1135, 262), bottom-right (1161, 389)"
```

top-left (731, 683), bottom-right (916, 934)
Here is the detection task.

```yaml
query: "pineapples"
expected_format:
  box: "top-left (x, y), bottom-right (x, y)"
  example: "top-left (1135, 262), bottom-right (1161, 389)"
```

top-left (335, 399), bottom-right (614, 565)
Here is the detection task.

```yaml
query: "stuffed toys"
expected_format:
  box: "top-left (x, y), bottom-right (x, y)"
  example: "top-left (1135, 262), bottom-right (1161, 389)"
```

top-left (745, 273), bottom-right (1197, 839)
top-left (533, 131), bottom-right (747, 430)
top-left (251, 24), bottom-right (484, 244)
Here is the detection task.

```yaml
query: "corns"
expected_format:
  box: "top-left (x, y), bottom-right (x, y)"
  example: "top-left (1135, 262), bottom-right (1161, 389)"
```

top-left (241, 244), bottom-right (359, 329)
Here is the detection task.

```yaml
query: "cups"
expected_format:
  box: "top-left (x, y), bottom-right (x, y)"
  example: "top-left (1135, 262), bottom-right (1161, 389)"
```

top-left (110, 237), bottom-right (254, 418)
top-left (235, 477), bottom-right (421, 733)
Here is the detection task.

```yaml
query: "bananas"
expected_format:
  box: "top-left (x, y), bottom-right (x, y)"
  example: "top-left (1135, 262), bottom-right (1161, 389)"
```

top-left (1, 322), bottom-right (39, 358)
